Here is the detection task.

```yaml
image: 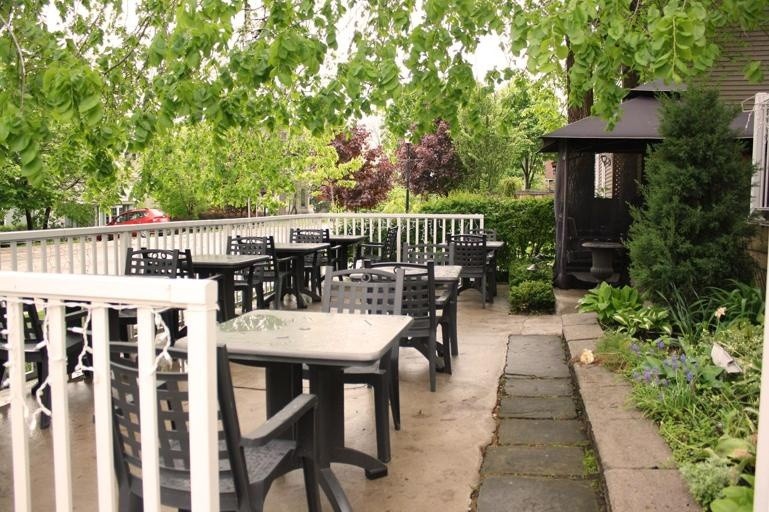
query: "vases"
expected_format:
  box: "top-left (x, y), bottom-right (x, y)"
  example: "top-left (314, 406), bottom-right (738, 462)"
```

top-left (581, 242), bottom-right (622, 289)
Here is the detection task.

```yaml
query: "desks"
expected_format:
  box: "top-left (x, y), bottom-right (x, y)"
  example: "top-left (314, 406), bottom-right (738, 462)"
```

top-left (439, 241), bottom-right (505, 303)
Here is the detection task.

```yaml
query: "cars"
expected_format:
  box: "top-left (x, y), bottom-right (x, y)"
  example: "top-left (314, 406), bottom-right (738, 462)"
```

top-left (95, 207), bottom-right (172, 242)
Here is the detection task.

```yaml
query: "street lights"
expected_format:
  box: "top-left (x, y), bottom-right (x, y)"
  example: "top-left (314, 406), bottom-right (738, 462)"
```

top-left (405, 129), bottom-right (412, 213)
top-left (260, 187), bottom-right (268, 215)
